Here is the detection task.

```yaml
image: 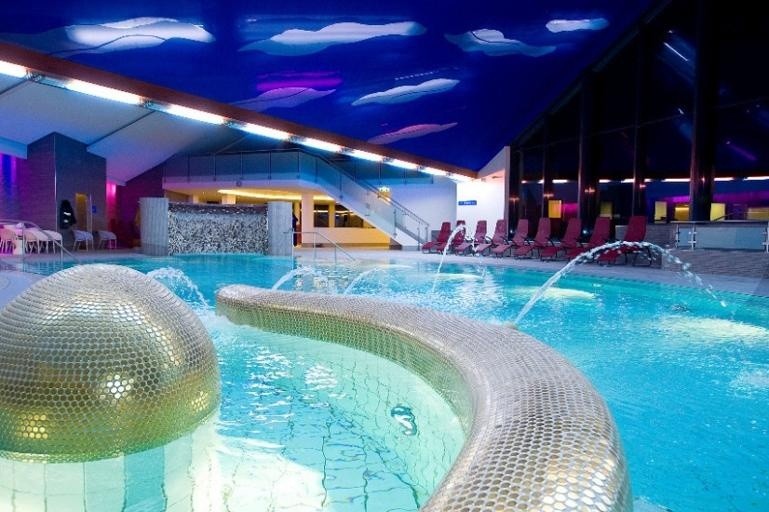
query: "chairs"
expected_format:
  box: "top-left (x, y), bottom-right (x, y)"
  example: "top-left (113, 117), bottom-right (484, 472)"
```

top-left (419, 215), bottom-right (653, 268)
top-left (0, 223), bottom-right (118, 254)
